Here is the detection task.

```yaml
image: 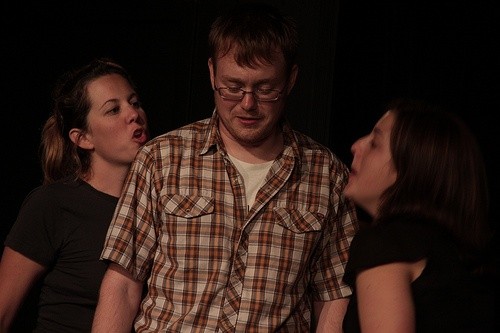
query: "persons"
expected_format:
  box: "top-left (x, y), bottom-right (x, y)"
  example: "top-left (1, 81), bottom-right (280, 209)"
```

top-left (1, 59), bottom-right (148, 333)
top-left (341, 91), bottom-right (490, 333)
top-left (91, 14), bottom-right (356, 333)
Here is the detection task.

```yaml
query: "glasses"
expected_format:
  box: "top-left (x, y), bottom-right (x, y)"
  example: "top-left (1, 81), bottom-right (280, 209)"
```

top-left (213, 78), bottom-right (290, 101)
